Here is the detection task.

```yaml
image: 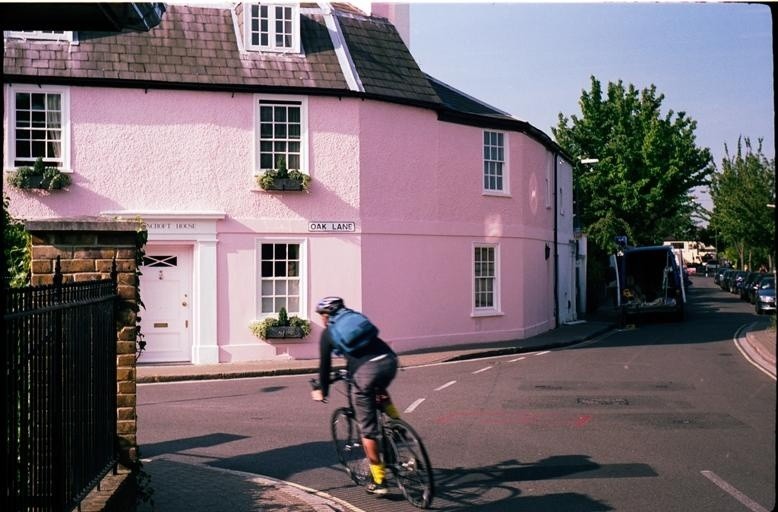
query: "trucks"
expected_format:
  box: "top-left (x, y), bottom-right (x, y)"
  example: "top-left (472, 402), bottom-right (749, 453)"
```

top-left (664, 240), bottom-right (717, 265)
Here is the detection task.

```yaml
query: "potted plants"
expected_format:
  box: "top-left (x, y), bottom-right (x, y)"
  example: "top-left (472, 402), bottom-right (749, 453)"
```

top-left (255, 153), bottom-right (313, 193)
top-left (251, 305), bottom-right (312, 341)
top-left (6, 157), bottom-right (74, 192)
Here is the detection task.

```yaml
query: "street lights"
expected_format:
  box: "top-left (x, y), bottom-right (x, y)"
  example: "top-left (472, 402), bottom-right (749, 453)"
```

top-left (576, 156), bottom-right (599, 228)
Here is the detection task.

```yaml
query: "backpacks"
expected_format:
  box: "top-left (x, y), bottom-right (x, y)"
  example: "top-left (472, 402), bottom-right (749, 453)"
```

top-left (327, 309), bottom-right (379, 353)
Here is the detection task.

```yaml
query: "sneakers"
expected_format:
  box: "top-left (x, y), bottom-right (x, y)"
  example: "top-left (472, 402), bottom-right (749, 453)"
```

top-left (365, 478), bottom-right (390, 495)
top-left (393, 419), bottom-right (406, 441)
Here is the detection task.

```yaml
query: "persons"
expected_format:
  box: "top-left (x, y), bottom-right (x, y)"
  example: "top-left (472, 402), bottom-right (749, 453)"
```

top-left (309, 294), bottom-right (412, 495)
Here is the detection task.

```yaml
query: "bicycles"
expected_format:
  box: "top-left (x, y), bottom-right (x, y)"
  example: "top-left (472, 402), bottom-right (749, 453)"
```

top-left (309, 371), bottom-right (433, 509)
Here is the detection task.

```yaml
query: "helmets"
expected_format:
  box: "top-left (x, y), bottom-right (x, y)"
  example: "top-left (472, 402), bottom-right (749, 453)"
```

top-left (315, 296), bottom-right (344, 314)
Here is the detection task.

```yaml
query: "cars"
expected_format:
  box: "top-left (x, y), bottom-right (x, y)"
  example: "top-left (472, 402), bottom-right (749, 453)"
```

top-left (702, 256), bottom-right (777, 314)
